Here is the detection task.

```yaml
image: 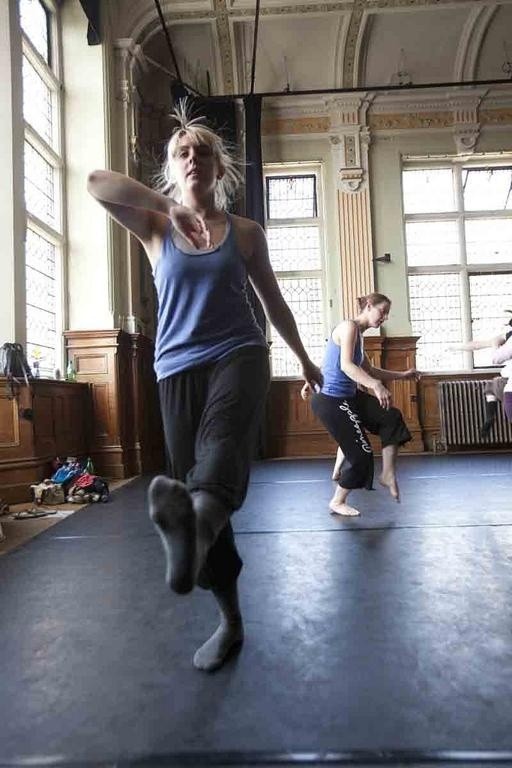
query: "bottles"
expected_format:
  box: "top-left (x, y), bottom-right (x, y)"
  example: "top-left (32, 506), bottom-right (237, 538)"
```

top-left (86, 458), bottom-right (94, 474)
top-left (66, 360), bottom-right (75, 381)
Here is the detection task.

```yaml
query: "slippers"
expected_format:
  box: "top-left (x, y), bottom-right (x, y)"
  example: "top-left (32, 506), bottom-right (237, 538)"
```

top-left (12, 504), bottom-right (58, 519)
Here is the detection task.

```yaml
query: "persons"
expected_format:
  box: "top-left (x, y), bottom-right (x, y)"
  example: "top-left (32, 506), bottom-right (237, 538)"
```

top-left (308, 293), bottom-right (423, 517)
top-left (88, 95), bottom-right (325, 673)
top-left (447, 319), bottom-right (511, 437)
top-left (490, 331), bottom-right (511, 426)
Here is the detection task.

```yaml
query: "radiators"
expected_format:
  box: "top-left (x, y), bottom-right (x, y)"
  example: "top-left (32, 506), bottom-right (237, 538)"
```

top-left (438, 381), bottom-right (512, 445)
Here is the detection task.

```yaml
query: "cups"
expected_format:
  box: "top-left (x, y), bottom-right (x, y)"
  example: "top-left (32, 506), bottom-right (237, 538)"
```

top-left (33, 361), bottom-right (40, 380)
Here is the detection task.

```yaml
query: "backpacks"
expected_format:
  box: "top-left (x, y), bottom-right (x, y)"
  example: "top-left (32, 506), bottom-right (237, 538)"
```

top-left (0, 342), bottom-right (33, 378)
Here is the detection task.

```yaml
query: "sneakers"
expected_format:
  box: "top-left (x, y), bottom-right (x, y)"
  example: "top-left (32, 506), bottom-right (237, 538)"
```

top-left (69, 493), bottom-right (108, 504)
